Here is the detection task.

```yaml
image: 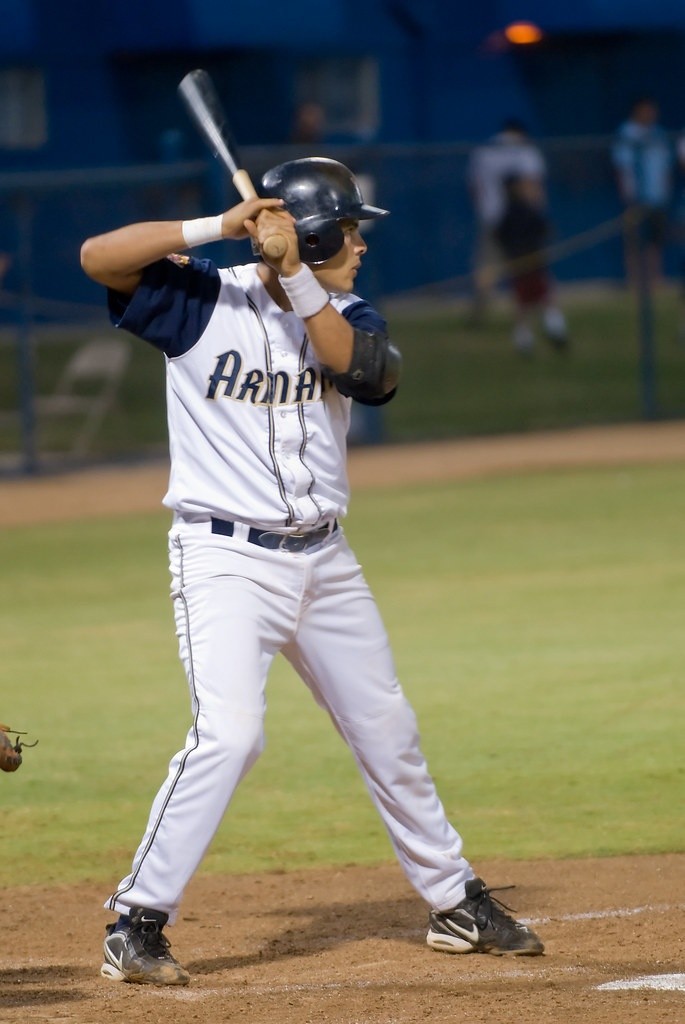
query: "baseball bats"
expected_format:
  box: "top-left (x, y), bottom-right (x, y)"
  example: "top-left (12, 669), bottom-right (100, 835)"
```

top-left (174, 69), bottom-right (288, 260)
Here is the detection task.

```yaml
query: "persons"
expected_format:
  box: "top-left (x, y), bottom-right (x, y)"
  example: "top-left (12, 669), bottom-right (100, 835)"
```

top-left (611, 101), bottom-right (685, 291)
top-left (464, 122), bottom-right (569, 364)
top-left (79, 158), bottom-right (545, 983)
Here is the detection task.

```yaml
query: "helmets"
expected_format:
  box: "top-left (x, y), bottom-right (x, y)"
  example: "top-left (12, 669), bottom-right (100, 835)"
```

top-left (251, 157), bottom-right (392, 265)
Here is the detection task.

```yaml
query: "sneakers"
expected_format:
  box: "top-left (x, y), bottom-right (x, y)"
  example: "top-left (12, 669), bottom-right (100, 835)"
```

top-left (100, 906), bottom-right (190, 985)
top-left (426, 877), bottom-right (544, 957)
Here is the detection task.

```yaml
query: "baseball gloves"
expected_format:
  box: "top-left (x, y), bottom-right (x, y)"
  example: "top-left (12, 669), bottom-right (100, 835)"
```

top-left (0, 722), bottom-right (40, 772)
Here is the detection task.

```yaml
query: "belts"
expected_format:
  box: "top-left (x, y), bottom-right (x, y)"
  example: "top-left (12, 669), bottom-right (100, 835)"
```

top-left (211, 516), bottom-right (338, 552)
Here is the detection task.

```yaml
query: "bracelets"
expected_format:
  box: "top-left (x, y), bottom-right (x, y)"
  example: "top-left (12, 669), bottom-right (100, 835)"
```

top-left (278, 263), bottom-right (331, 319)
top-left (181, 213), bottom-right (224, 249)
